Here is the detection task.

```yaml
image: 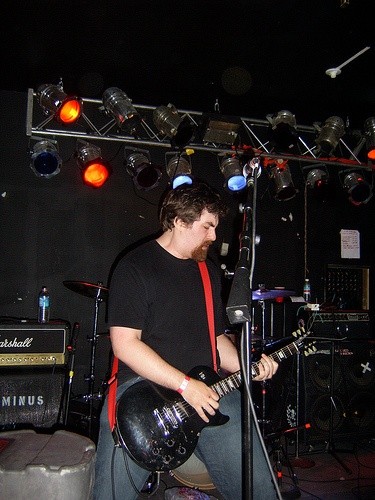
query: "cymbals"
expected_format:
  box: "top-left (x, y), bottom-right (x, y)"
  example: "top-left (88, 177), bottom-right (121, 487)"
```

top-left (62, 280), bottom-right (108, 302)
top-left (252, 289), bottom-right (297, 300)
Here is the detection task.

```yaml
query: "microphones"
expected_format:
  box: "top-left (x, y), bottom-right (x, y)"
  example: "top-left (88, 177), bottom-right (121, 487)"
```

top-left (242, 157), bottom-right (262, 180)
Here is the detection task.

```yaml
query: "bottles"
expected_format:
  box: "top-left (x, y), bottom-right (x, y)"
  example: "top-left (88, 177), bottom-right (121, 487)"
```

top-left (37, 286), bottom-right (50, 323)
top-left (303, 279), bottom-right (311, 303)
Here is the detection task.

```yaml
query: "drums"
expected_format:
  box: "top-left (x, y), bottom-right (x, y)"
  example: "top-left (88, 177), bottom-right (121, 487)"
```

top-left (168, 452), bottom-right (217, 491)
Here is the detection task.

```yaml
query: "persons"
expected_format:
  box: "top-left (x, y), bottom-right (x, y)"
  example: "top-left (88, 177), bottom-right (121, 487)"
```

top-left (88, 180), bottom-right (280, 500)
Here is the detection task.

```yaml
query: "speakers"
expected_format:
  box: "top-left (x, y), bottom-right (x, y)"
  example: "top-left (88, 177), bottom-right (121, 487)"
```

top-left (298, 337), bottom-right (375, 445)
top-left (0, 369), bottom-right (67, 432)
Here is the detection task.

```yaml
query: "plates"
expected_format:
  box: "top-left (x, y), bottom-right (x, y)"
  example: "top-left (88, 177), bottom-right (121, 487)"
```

top-left (275, 287), bottom-right (285, 290)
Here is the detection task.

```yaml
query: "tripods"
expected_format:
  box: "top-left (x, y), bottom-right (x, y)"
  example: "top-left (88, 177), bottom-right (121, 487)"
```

top-left (265, 357), bottom-right (300, 491)
top-left (286, 313), bottom-right (357, 476)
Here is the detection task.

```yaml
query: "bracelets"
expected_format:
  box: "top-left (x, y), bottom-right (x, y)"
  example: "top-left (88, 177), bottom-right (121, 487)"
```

top-left (177, 375), bottom-right (190, 394)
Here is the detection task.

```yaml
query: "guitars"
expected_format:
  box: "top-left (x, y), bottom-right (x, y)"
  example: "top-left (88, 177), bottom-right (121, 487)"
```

top-left (114, 327), bottom-right (319, 473)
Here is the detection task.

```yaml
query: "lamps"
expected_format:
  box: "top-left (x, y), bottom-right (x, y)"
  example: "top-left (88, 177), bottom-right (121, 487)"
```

top-left (28, 84), bottom-right (375, 207)
